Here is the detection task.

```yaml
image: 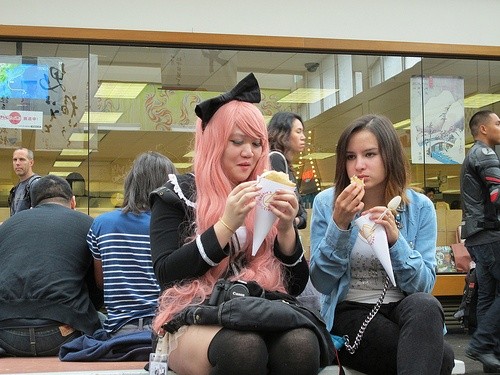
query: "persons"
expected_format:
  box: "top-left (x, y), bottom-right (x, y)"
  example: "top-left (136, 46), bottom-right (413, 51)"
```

top-left (460, 112), bottom-right (500, 374)
top-left (87, 151), bottom-right (177, 334)
top-left (267, 111), bottom-right (307, 230)
top-left (309, 115), bottom-right (456, 375)
top-left (420, 187), bottom-right (435, 203)
top-left (144, 72), bottom-right (320, 375)
top-left (0, 175), bottom-right (103, 357)
top-left (8, 148), bottom-right (42, 215)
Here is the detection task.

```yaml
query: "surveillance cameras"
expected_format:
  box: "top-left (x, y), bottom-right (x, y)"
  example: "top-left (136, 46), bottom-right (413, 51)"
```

top-left (305, 62), bottom-right (319, 72)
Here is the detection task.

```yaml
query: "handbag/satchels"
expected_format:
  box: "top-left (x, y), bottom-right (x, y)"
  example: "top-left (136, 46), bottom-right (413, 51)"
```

top-left (185, 281), bottom-right (337, 368)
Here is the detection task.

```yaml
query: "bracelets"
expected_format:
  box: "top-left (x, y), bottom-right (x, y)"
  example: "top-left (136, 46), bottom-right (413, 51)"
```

top-left (220, 218), bottom-right (235, 234)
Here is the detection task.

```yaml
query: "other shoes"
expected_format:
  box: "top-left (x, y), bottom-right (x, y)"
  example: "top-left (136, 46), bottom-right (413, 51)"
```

top-left (455, 310), bottom-right (477, 327)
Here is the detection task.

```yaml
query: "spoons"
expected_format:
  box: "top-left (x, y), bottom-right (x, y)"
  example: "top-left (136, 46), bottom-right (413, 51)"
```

top-left (370, 195), bottom-right (402, 233)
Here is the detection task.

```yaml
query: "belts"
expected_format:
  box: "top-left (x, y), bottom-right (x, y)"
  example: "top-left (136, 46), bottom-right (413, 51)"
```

top-left (130, 317), bottom-right (151, 326)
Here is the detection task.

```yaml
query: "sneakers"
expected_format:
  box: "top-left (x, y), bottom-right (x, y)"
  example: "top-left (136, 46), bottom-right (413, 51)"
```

top-left (465, 345), bottom-right (500, 371)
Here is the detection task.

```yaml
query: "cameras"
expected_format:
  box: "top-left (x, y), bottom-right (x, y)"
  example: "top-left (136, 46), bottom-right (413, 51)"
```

top-left (208, 277), bottom-right (266, 307)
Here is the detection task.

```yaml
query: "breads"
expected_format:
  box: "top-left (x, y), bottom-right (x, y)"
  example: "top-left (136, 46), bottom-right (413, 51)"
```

top-left (350, 175), bottom-right (365, 195)
top-left (261, 170), bottom-right (297, 187)
